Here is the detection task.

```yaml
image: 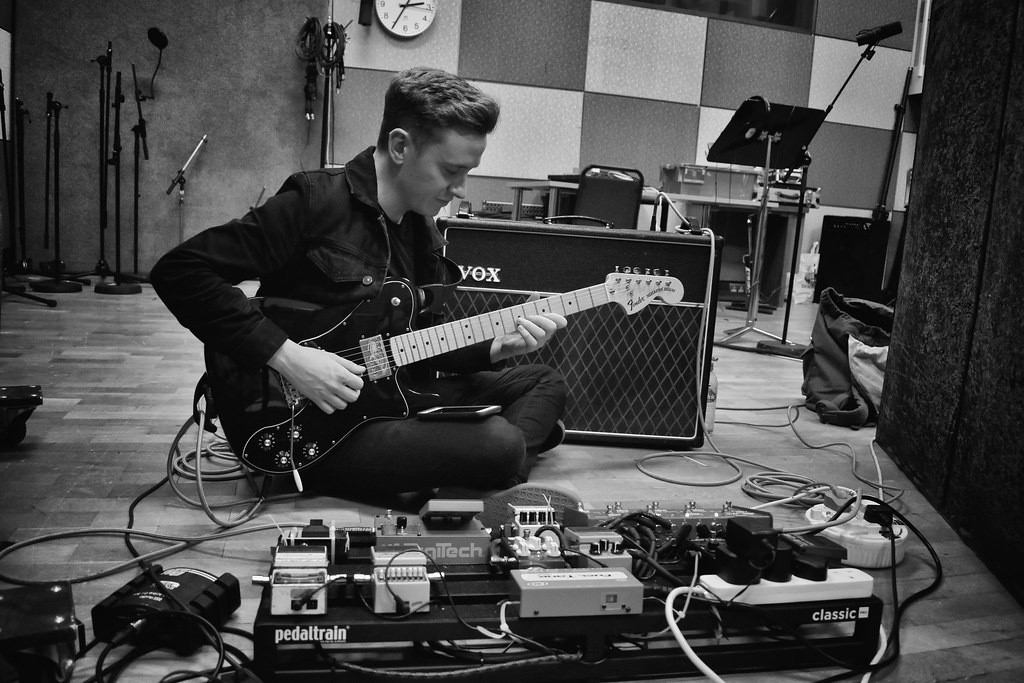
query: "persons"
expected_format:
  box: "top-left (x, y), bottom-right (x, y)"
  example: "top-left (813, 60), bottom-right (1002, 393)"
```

top-left (148, 66), bottom-right (570, 516)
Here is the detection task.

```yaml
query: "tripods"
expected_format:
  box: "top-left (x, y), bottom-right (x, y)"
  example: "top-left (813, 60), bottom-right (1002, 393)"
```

top-left (710, 137), bottom-right (799, 350)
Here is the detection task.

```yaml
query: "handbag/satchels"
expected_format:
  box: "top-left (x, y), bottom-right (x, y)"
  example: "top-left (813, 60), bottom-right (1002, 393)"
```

top-left (800, 282), bottom-right (899, 426)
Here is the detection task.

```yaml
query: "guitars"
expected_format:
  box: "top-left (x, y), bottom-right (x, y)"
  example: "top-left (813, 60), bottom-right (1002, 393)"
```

top-left (220, 263), bottom-right (685, 476)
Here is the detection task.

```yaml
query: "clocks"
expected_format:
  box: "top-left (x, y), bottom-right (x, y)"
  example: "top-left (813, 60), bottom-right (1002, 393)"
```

top-left (374, 0), bottom-right (437, 38)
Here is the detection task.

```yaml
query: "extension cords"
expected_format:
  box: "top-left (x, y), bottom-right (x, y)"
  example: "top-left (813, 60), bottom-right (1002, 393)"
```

top-left (804, 496), bottom-right (910, 568)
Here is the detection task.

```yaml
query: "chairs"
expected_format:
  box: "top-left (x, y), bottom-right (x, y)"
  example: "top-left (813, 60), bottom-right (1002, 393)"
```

top-left (570, 164), bottom-right (644, 230)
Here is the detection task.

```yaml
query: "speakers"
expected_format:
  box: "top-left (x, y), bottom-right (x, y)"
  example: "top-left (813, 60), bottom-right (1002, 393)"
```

top-left (433, 215), bottom-right (722, 448)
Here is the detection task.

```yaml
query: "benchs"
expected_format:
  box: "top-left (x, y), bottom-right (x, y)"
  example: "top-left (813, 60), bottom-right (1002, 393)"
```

top-left (507, 180), bottom-right (811, 313)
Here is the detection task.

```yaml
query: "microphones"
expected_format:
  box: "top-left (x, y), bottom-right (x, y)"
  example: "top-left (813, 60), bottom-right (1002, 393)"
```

top-left (856, 21), bottom-right (903, 45)
